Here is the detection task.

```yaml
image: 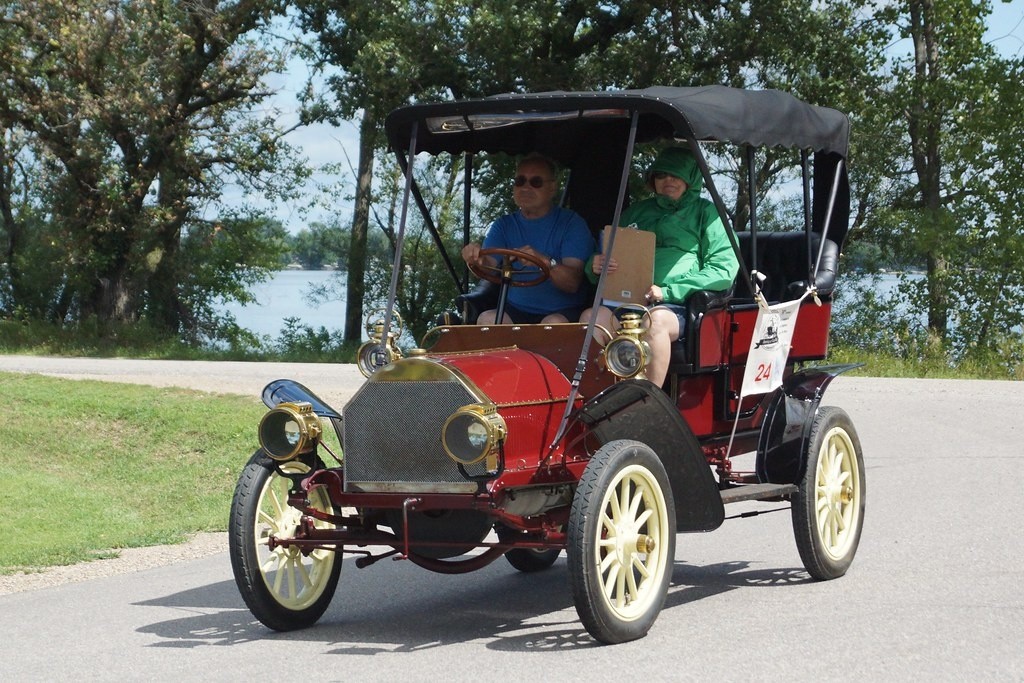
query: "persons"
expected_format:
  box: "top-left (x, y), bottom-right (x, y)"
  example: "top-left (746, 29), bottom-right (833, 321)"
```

top-left (461, 156), bottom-right (594, 326)
top-left (577, 144), bottom-right (740, 389)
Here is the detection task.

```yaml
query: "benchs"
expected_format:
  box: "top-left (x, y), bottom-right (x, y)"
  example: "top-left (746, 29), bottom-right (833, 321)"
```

top-left (733, 230), bottom-right (842, 303)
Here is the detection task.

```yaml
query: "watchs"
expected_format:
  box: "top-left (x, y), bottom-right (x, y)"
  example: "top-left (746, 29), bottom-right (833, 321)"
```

top-left (548, 258), bottom-right (558, 271)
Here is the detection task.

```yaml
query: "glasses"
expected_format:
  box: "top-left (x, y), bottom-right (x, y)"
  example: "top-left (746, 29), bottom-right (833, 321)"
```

top-left (654, 172), bottom-right (680, 179)
top-left (514, 176), bottom-right (554, 188)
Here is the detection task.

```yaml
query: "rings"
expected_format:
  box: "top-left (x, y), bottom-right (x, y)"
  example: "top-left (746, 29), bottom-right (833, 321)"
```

top-left (644, 294), bottom-right (650, 299)
top-left (600, 266), bottom-right (602, 269)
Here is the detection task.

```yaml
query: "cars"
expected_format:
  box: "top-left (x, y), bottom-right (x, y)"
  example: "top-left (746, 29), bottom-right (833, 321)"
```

top-left (228, 83), bottom-right (866, 645)
